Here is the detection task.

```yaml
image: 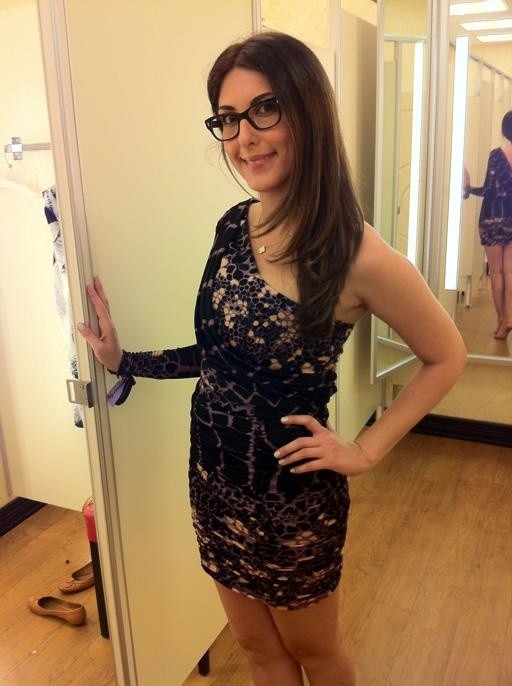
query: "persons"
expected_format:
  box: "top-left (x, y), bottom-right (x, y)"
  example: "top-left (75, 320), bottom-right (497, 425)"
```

top-left (463, 110), bottom-right (512, 339)
top-left (76, 30), bottom-right (470, 686)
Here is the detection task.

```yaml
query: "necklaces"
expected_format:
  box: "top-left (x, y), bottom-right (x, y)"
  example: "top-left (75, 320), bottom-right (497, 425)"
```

top-left (255, 214), bottom-right (294, 255)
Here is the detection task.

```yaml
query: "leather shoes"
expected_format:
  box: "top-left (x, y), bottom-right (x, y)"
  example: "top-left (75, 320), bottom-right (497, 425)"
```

top-left (29, 595), bottom-right (85, 624)
top-left (58, 560), bottom-right (95, 592)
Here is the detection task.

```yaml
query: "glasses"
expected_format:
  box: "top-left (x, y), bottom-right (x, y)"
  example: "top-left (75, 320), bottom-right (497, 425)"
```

top-left (205, 96), bottom-right (282, 141)
